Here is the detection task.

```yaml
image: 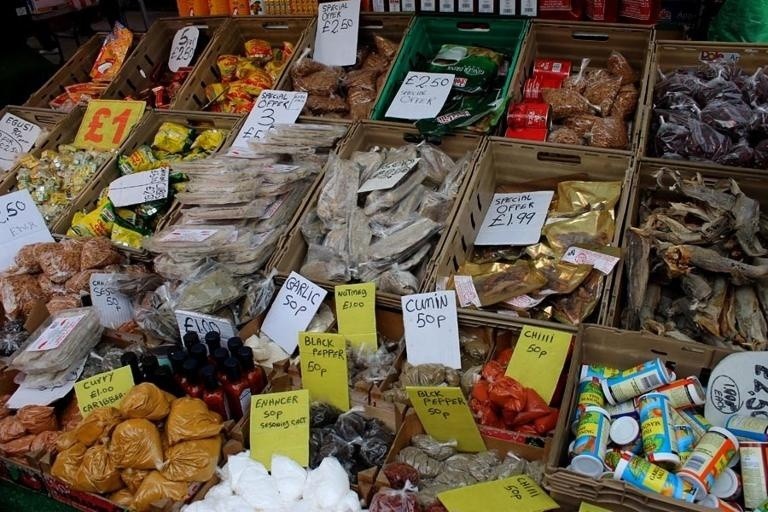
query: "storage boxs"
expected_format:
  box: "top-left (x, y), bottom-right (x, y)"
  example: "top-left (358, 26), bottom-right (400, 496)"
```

top-left (23, 32), bottom-right (145, 115)
top-left (372, 11), bottom-right (529, 131)
top-left (99, 15), bottom-right (229, 111)
top-left (376, 409), bottom-right (553, 512)
top-left (384, 318), bottom-right (495, 404)
top-left (51, 108), bottom-right (242, 263)
top-left (0, 365), bottom-right (45, 498)
top-left (151, 113), bottom-right (355, 278)
top-left (235, 283), bottom-right (406, 391)
top-left (493, 17), bottom-right (657, 154)
top-left (368, 488), bottom-right (386, 512)
top-left (169, 16), bottom-right (315, 117)
top-left (230, 372), bottom-right (407, 484)
top-left (542, 323), bottom-right (768, 511)
top-left (183, 439), bottom-right (372, 511)
top-left (467, 331), bottom-right (576, 462)
top-left (422, 137), bottom-right (637, 337)
top-left (273, 13), bottom-right (415, 122)
top-left (270, 119), bottom-right (488, 311)
top-left (637, 40), bottom-right (768, 174)
top-left (0, 102), bottom-right (151, 232)
top-left (605, 158), bottom-right (768, 369)
top-left (43, 389), bottom-right (227, 512)
top-left (1, 105), bottom-right (67, 198)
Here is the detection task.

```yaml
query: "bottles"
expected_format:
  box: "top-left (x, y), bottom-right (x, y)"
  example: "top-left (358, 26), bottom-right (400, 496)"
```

top-left (569, 356), bottom-right (768, 512)
top-left (120, 331), bottom-right (268, 421)
